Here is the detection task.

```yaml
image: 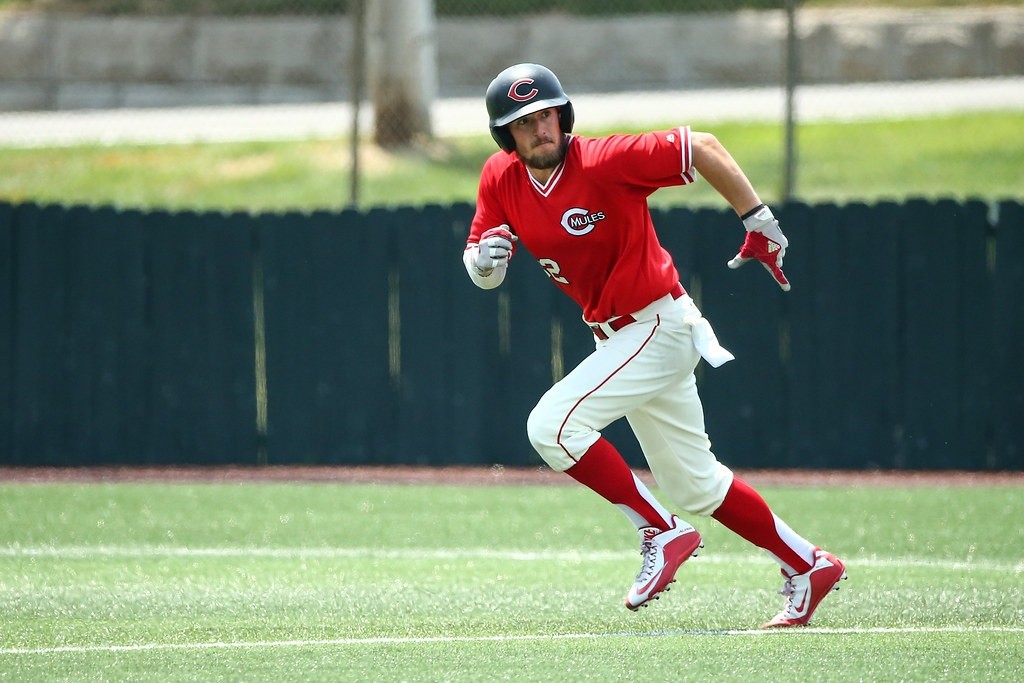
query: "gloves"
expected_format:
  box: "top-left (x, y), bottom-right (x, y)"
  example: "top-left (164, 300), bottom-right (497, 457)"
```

top-left (473, 224), bottom-right (518, 277)
top-left (728, 204), bottom-right (791, 291)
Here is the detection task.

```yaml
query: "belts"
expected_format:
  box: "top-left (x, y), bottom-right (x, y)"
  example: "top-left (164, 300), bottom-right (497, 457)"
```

top-left (594, 283), bottom-right (686, 341)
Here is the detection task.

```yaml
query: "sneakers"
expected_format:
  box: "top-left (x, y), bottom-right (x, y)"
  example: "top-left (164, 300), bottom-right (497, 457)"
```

top-left (763, 545), bottom-right (847, 627)
top-left (625, 514), bottom-right (705, 613)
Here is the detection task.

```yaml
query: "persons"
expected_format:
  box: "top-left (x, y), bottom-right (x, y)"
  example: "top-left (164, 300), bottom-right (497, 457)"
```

top-left (462, 63), bottom-right (849, 628)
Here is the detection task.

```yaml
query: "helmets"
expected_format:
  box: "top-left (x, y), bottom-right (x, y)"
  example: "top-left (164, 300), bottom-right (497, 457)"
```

top-left (486, 62), bottom-right (577, 154)
